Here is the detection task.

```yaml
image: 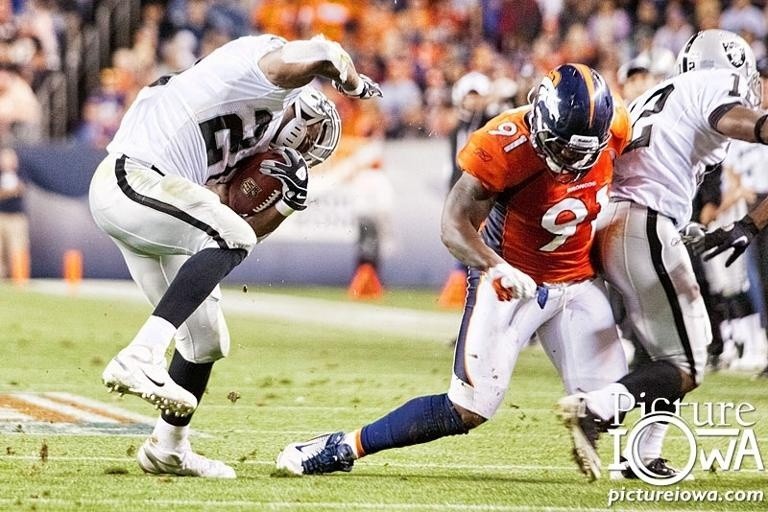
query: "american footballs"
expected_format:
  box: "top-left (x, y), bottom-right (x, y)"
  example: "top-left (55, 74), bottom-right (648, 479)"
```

top-left (229, 150), bottom-right (287, 216)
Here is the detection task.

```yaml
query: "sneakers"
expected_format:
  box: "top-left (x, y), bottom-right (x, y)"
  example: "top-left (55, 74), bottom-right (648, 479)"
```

top-left (612, 456), bottom-right (695, 483)
top-left (278, 431), bottom-right (354, 476)
top-left (102, 354), bottom-right (197, 418)
top-left (138, 437), bottom-right (235, 480)
top-left (553, 397), bottom-right (606, 479)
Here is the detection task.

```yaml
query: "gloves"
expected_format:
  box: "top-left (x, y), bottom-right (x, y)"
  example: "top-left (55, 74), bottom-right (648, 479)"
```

top-left (488, 260), bottom-right (536, 300)
top-left (261, 148), bottom-right (309, 214)
top-left (332, 72), bottom-right (384, 102)
top-left (689, 214), bottom-right (761, 267)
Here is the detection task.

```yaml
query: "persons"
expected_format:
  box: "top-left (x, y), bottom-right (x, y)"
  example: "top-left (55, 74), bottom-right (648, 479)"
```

top-left (0, 0), bottom-right (768, 479)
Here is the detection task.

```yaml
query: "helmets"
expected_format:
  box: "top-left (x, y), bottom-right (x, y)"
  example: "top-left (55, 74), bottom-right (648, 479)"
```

top-left (528, 65), bottom-right (612, 173)
top-left (273, 86), bottom-right (341, 168)
top-left (678, 29), bottom-right (763, 106)
